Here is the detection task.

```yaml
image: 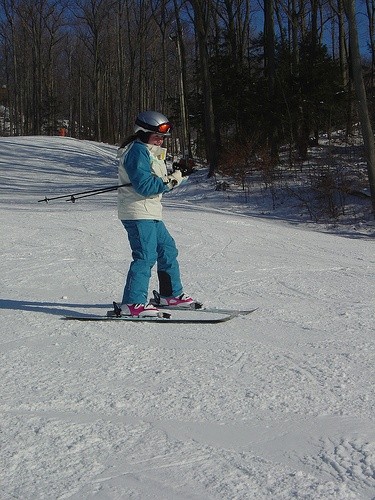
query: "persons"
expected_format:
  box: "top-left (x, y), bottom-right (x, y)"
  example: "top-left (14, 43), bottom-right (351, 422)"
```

top-left (110, 111), bottom-right (203, 317)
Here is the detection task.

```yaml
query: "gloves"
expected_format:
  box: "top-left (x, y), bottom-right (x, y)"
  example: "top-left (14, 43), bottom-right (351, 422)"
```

top-left (178, 168), bottom-right (191, 187)
top-left (167, 162), bottom-right (183, 189)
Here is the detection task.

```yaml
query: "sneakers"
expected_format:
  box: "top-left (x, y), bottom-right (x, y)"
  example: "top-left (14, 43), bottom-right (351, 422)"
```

top-left (120, 302), bottom-right (161, 316)
top-left (159, 293), bottom-right (203, 308)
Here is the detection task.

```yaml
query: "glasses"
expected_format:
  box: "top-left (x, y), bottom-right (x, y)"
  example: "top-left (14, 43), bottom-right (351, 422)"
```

top-left (158, 123), bottom-right (172, 133)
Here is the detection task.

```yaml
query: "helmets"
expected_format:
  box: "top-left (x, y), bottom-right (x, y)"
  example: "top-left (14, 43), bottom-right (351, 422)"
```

top-left (133, 111), bottom-right (172, 137)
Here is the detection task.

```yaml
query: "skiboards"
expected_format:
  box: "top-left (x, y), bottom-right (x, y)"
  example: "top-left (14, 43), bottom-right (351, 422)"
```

top-left (65, 313), bottom-right (237, 323)
top-left (96, 304), bottom-right (259, 316)
